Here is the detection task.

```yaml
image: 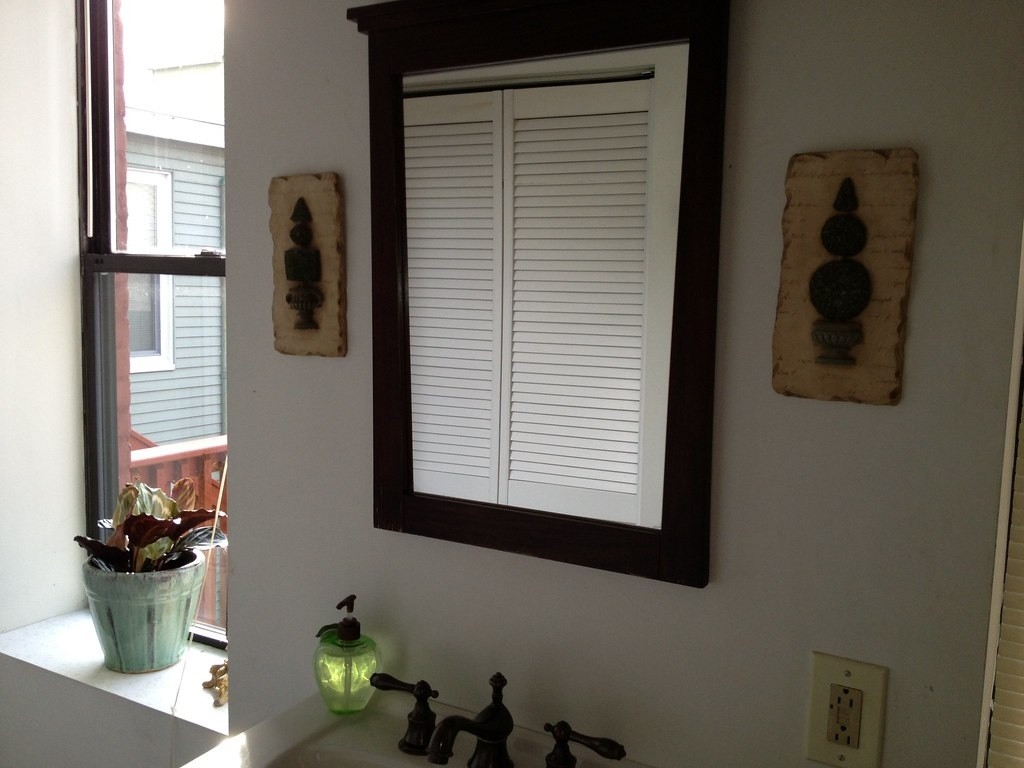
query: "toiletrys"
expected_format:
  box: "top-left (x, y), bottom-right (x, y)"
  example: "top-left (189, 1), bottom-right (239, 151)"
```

top-left (311, 594), bottom-right (384, 715)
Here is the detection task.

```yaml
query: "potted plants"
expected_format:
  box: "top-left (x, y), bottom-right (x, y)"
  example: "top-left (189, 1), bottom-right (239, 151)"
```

top-left (73, 476), bottom-right (227, 674)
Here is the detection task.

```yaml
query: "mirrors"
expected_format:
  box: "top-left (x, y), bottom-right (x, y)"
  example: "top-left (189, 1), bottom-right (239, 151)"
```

top-left (342, 0), bottom-right (727, 589)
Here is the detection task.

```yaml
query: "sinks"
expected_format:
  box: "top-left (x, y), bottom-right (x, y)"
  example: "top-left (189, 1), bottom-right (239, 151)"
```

top-left (182, 682), bottom-right (656, 768)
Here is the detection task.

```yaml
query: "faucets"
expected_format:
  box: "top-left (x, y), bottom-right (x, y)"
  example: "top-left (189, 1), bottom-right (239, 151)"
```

top-left (425, 671), bottom-right (515, 768)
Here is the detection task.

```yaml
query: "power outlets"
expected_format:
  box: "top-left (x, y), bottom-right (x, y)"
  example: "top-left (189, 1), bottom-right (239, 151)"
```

top-left (803, 650), bottom-right (890, 768)
